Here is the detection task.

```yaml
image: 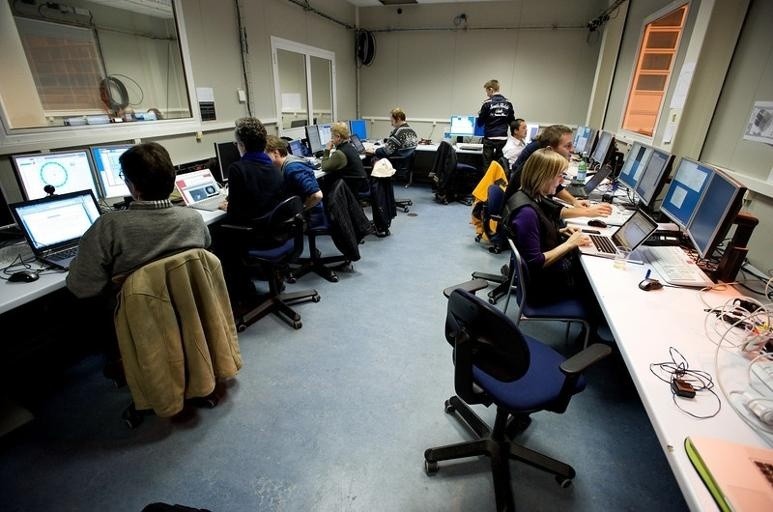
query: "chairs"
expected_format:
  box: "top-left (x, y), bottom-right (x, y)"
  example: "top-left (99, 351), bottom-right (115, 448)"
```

top-left (288, 182), bottom-right (354, 283)
top-left (359, 174), bottom-right (397, 237)
top-left (472, 185), bottom-right (531, 304)
top-left (433, 142), bottom-right (477, 205)
top-left (498, 157), bottom-right (510, 177)
top-left (115, 248), bottom-right (245, 430)
top-left (425, 291), bottom-right (612, 512)
top-left (388, 147), bottom-right (415, 188)
top-left (504, 239), bottom-right (600, 353)
top-left (222, 195), bottom-right (319, 330)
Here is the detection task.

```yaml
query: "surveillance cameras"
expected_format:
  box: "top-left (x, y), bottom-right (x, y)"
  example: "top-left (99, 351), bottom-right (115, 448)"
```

top-left (44, 184), bottom-right (59, 197)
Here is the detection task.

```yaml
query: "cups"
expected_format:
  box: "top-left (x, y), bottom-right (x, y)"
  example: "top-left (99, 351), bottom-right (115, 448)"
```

top-left (613, 246), bottom-right (632, 270)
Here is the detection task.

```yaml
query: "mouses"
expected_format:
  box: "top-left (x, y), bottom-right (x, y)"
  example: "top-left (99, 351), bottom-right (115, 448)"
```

top-left (639, 279), bottom-right (663, 290)
top-left (587, 219), bottom-right (607, 228)
top-left (9, 272), bottom-right (39, 282)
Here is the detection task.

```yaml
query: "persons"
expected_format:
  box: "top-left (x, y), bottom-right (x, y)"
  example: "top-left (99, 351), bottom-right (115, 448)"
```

top-left (503, 118), bottom-right (528, 171)
top-left (322, 124), bottom-right (368, 193)
top-left (66, 142), bottom-right (212, 300)
top-left (505, 125), bottom-right (612, 222)
top-left (512, 137), bottom-right (542, 180)
top-left (219, 116), bottom-right (285, 251)
top-left (263, 135), bottom-right (323, 231)
top-left (505, 147), bottom-right (612, 350)
top-left (476, 80), bottom-right (515, 174)
top-left (364, 108), bottom-right (418, 174)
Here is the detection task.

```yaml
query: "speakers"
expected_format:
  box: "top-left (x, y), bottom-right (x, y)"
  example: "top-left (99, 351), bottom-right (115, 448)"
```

top-left (358, 31), bottom-right (374, 65)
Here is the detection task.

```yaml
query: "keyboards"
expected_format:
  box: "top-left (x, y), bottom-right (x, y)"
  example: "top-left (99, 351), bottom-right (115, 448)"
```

top-left (455, 142), bottom-right (485, 151)
top-left (750, 361), bottom-right (773, 392)
top-left (313, 162), bottom-right (322, 170)
top-left (643, 246), bottom-right (716, 287)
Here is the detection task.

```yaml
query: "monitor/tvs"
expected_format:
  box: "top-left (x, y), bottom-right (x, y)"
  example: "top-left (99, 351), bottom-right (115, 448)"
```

top-left (317, 124), bottom-right (331, 145)
top-left (349, 120), bottom-right (368, 142)
top-left (573, 124), bottom-right (676, 208)
top-left (475, 117), bottom-right (485, 137)
top-left (11, 149), bottom-right (102, 201)
top-left (659, 155), bottom-right (716, 231)
top-left (304, 125), bottom-right (322, 154)
top-left (85, 116), bottom-right (110, 125)
top-left (88, 144), bottom-right (133, 198)
top-left (124, 113), bottom-right (157, 121)
top-left (686, 168), bottom-right (747, 259)
top-left (449, 115), bottom-right (475, 135)
top-left (67, 117), bottom-right (87, 126)
top-left (214, 141), bottom-right (243, 183)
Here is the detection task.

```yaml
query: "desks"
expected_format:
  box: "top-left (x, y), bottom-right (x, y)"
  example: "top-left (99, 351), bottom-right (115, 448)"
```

top-left (415, 143), bottom-right (496, 155)
top-left (0, 140), bottom-right (387, 436)
top-left (554, 161), bottom-right (773, 512)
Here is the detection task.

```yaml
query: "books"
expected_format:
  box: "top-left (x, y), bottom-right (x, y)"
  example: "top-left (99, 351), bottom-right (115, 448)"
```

top-left (685, 436), bottom-right (773, 512)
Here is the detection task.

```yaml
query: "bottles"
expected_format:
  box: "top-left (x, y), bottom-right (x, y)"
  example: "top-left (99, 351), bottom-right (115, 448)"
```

top-left (576, 151), bottom-right (587, 182)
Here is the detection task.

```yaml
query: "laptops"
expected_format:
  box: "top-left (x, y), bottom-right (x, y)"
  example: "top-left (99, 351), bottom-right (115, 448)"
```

top-left (349, 134), bottom-right (374, 156)
top-left (565, 165), bottom-right (612, 199)
top-left (288, 139), bottom-right (316, 161)
top-left (578, 208), bottom-right (659, 260)
top-left (175, 168), bottom-right (227, 212)
top-left (7, 188), bottom-right (102, 270)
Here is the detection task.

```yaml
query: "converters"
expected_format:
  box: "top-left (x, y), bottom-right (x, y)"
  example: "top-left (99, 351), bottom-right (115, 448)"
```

top-left (671, 381), bottom-right (696, 398)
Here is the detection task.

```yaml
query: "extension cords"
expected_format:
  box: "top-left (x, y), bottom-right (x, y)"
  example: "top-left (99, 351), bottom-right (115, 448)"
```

top-left (740, 393), bottom-right (773, 424)
